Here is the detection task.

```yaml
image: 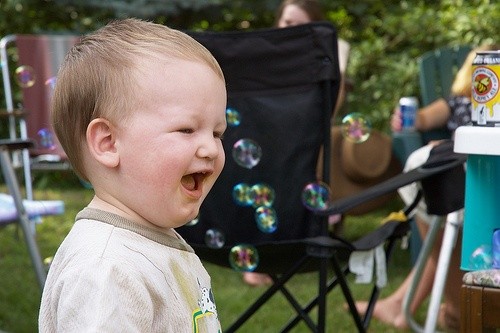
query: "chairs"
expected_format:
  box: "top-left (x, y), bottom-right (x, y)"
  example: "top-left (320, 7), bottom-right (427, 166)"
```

top-left (0, 34), bottom-right (98, 238)
top-left (182, 21), bottom-right (466, 332)
top-left (0, 111), bottom-right (66, 290)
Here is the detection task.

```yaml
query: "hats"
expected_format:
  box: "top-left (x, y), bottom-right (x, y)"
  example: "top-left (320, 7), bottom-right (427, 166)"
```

top-left (316, 125), bottom-right (404, 216)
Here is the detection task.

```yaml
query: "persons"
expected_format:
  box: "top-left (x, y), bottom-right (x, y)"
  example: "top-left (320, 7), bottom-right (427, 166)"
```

top-left (37, 18), bottom-right (227, 333)
top-left (343, 35), bottom-right (500, 330)
top-left (242, 0), bottom-right (351, 285)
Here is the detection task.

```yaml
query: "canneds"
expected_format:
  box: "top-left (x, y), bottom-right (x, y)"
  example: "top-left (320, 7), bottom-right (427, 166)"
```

top-left (470, 50), bottom-right (500, 127)
top-left (398, 97), bottom-right (418, 133)
